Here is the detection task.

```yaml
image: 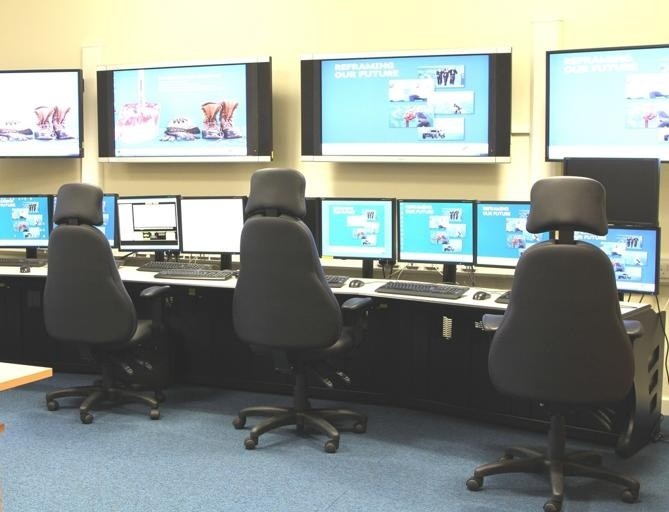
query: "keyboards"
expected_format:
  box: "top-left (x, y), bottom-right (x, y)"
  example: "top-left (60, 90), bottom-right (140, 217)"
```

top-left (324, 274), bottom-right (349, 288)
top-left (137, 259), bottom-right (222, 272)
top-left (1, 255), bottom-right (48, 267)
top-left (494, 290), bottom-right (514, 304)
top-left (374, 281), bottom-right (469, 299)
top-left (154, 265), bottom-right (232, 281)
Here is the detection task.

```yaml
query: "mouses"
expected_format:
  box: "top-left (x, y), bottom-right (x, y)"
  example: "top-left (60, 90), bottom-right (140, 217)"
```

top-left (472, 291), bottom-right (491, 300)
top-left (349, 280), bottom-right (364, 288)
top-left (20, 263), bottom-right (31, 273)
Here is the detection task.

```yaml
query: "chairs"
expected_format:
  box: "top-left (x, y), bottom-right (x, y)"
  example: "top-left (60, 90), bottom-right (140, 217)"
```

top-left (41, 184), bottom-right (171, 423)
top-left (232, 168), bottom-right (368, 453)
top-left (467, 177), bottom-right (639, 507)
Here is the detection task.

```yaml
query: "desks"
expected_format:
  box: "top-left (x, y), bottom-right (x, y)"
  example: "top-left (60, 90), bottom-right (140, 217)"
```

top-left (1, 264), bottom-right (664, 459)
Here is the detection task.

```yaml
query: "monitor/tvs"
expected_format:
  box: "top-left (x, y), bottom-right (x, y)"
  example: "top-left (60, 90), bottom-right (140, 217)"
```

top-left (396, 199), bottom-right (475, 284)
top-left (95, 55), bottom-right (274, 165)
top-left (319, 197), bottom-right (395, 277)
top-left (572, 226), bottom-right (661, 301)
top-left (52, 192), bottom-right (120, 249)
top-left (299, 47), bottom-right (511, 164)
top-left (562, 157), bottom-right (660, 226)
top-left (475, 201), bottom-right (554, 269)
top-left (0, 193), bottom-right (53, 259)
top-left (114, 195), bottom-right (180, 263)
top-left (178, 195), bottom-right (247, 271)
top-left (0, 70), bottom-right (85, 158)
top-left (545, 44), bottom-right (669, 162)
